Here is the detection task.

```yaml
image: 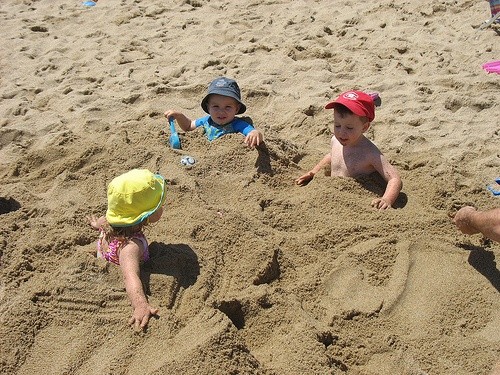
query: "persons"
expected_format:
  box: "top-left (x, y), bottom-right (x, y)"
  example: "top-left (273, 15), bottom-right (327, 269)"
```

top-left (295, 89), bottom-right (403, 209)
top-left (452, 206), bottom-right (500, 243)
top-left (163, 77), bottom-right (263, 148)
top-left (84, 168), bottom-right (168, 333)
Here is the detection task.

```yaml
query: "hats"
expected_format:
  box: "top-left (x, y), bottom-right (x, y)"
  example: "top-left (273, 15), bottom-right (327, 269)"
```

top-left (201, 77), bottom-right (247, 116)
top-left (105, 168), bottom-right (167, 228)
top-left (324, 90), bottom-right (375, 123)
